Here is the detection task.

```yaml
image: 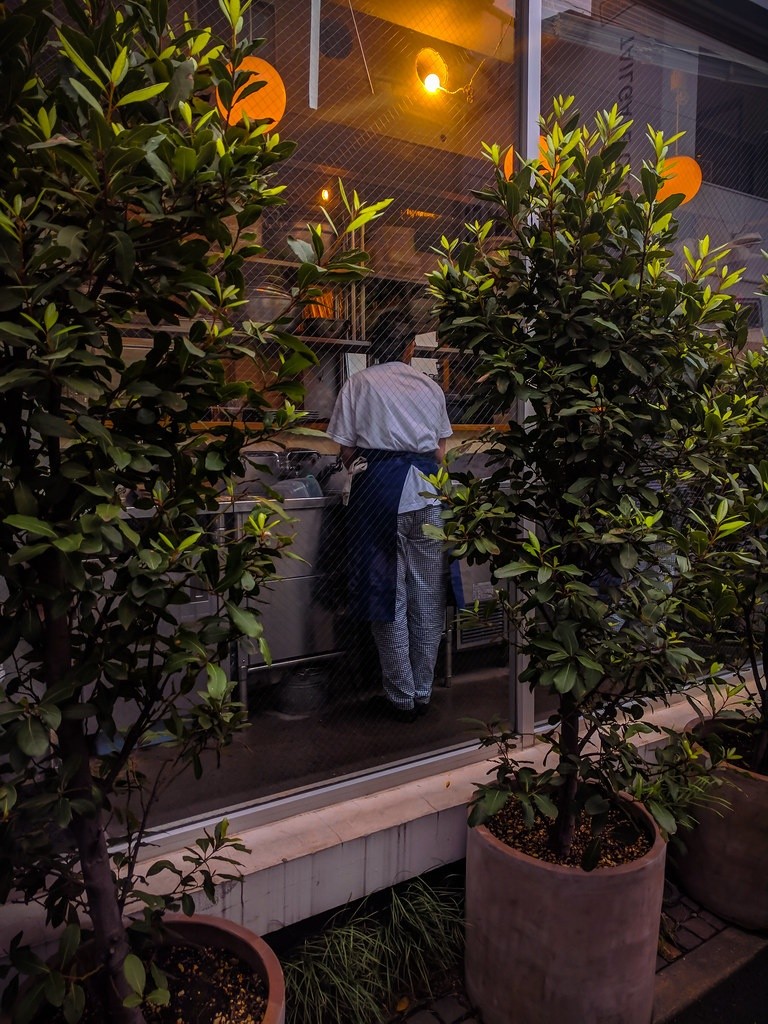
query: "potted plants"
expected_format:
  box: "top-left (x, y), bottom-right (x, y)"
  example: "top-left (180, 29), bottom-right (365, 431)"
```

top-left (683, 327), bottom-right (768, 936)
top-left (419, 96), bottom-right (768, 1024)
top-left (0, 1), bottom-right (396, 1024)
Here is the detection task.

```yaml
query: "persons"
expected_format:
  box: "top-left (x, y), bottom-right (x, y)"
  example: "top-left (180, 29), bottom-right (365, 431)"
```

top-left (324, 312), bottom-right (465, 723)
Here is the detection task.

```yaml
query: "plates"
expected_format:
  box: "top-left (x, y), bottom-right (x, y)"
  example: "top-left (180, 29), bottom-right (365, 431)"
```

top-left (264, 408), bottom-right (321, 424)
top-left (248, 275), bottom-right (292, 296)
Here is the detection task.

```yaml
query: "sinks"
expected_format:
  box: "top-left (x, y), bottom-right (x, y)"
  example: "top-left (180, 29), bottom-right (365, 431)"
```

top-left (261, 490), bottom-right (343, 513)
top-left (215, 492), bottom-right (260, 512)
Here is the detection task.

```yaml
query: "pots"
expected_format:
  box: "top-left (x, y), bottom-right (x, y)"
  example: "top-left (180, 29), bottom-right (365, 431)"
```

top-left (277, 666), bottom-right (328, 714)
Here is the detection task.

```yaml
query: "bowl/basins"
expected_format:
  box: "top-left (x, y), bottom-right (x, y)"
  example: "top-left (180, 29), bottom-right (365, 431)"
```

top-left (246, 295), bottom-right (301, 324)
top-left (305, 317), bottom-right (351, 340)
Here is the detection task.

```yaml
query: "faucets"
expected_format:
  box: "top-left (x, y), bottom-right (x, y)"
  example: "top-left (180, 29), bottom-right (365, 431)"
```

top-left (285, 450), bottom-right (322, 470)
top-left (241, 451), bottom-right (280, 469)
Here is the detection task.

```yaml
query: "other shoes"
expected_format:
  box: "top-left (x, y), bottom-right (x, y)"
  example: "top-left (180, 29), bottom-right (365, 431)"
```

top-left (341, 694), bottom-right (431, 723)
